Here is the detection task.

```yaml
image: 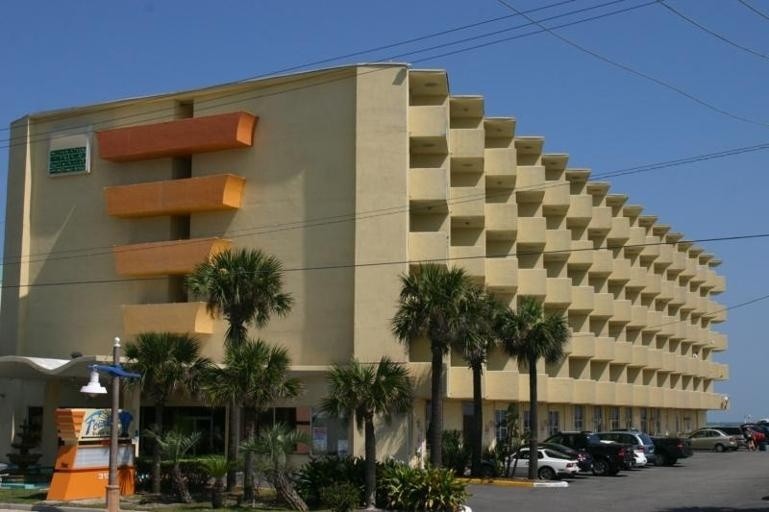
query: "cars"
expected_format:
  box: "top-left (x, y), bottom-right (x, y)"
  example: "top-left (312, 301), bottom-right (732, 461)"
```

top-left (499, 425), bottom-right (745, 481)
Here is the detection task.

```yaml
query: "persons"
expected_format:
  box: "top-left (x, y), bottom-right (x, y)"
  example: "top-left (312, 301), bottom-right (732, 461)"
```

top-left (692, 351), bottom-right (698, 359)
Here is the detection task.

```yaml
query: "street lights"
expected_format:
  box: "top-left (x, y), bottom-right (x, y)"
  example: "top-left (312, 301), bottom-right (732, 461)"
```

top-left (81, 336), bottom-right (121, 511)
